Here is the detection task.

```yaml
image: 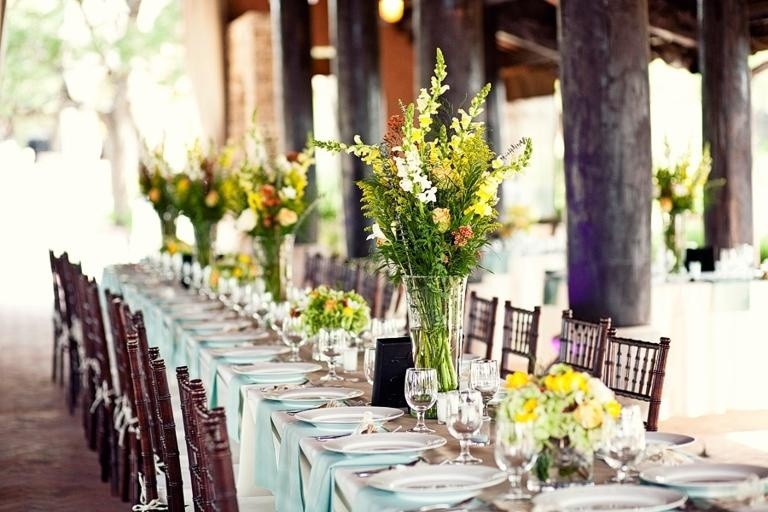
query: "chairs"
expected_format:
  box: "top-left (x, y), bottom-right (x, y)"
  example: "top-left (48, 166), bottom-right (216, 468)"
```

top-left (47, 246), bottom-right (766, 511)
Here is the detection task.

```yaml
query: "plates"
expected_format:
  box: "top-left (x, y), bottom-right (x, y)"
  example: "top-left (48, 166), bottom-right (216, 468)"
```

top-left (527, 486), bottom-right (689, 511)
top-left (636, 461), bottom-right (768, 500)
top-left (459, 353), bottom-right (481, 389)
top-left (365, 463), bottom-right (510, 505)
top-left (293, 405), bottom-right (404, 430)
top-left (322, 431), bottom-right (449, 464)
top-left (593, 431), bottom-right (695, 462)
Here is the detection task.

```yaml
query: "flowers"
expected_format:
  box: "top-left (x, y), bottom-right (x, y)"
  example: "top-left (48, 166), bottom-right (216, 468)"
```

top-left (645, 136), bottom-right (717, 243)
top-left (212, 98), bottom-right (319, 287)
top-left (163, 131), bottom-right (232, 219)
top-left (325, 38), bottom-right (533, 389)
top-left (134, 141), bottom-right (201, 215)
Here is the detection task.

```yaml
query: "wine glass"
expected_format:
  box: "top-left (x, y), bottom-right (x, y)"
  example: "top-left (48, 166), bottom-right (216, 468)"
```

top-left (443, 390), bottom-right (483, 463)
top-left (468, 359), bottom-right (502, 421)
top-left (493, 405), bottom-right (647, 501)
top-left (404, 366), bottom-right (440, 431)
top-left (110, 262), bottom-right (404, 425)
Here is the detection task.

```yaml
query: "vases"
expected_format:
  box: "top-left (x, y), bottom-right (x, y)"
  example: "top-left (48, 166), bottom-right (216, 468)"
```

top-left (160, 214), bottom-right (177, 244)
top-left (660, 212), bottom-right (687, 279)
top-left (188, 215), bottom-right (219, 267)
